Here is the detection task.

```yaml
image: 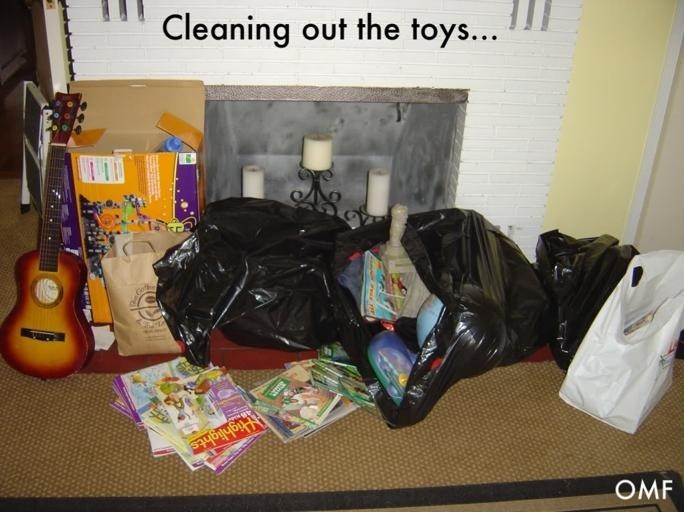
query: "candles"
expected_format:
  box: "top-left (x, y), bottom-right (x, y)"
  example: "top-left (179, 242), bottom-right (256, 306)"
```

top-left (239, 133), bottom-right (393, 217)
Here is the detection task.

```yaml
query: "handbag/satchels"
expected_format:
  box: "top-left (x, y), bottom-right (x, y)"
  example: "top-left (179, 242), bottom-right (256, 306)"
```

top-left (558, 250), bottom-right (684, 435)
top-left (100, 219), bottom-right (192, 357)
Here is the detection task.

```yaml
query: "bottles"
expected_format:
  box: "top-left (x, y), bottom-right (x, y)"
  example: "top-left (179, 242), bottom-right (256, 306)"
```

top-left (150, 136), bottom-right (183, 154)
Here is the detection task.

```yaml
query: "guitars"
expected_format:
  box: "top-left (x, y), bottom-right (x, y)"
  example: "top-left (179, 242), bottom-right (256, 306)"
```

top-left (0, 92), bottom-right (95, 378)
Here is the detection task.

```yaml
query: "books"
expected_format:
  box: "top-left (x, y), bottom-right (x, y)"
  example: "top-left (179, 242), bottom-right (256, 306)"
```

top-left (360, 250), bottom-right (408, 323)
top-left (110, 341), bottom-right (382, 475)
